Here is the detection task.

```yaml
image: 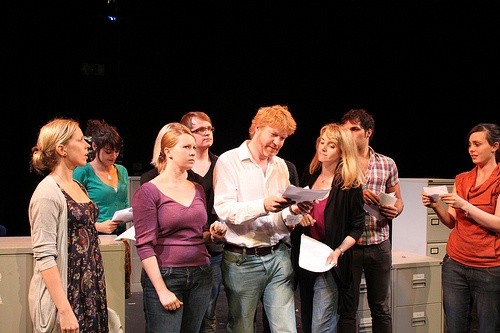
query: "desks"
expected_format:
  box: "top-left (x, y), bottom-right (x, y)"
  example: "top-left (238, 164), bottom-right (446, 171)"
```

top-left (0, 236), bottom-right (126, 333)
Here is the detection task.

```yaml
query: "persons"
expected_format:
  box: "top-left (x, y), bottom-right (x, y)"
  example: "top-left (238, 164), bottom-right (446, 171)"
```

top-left (212, 104), bottom-right (320, 333)
top-left (28, 119), bottom-right (110, 333)
top-left (132, 123), bottom-right (229, 333)
top-left (421, 123), bottom-right (500, 333)
top-left (181, 110), bottom-right (229, 333)
top-left (294, 109), bottom-right (405, 333)
top-left (72, 119), bottom-right (130, 236)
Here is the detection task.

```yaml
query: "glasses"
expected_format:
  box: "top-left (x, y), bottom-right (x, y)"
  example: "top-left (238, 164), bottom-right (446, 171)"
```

top-left (468, 142), bottom-right (488, 148)
top-left (191, 127), bottom-right (215, 134)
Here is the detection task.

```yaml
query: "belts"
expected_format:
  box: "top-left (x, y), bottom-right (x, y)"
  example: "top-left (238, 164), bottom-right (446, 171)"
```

top-left (223, 240), bottom-right (282, 257)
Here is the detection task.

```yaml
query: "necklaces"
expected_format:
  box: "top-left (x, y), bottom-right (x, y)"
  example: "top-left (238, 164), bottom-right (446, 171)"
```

top-left (107, 174), bottom-right (112, 181)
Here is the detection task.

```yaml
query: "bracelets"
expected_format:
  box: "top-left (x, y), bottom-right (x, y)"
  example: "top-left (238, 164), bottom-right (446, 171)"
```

top-left (464, 201), bottom-right (469, 216)
top-left (336, 247), bottom-right (344, 256)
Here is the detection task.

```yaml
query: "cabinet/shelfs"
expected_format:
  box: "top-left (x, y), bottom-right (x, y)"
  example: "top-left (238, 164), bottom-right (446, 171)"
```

top-left (426, 181), bottom-right (458, 255)
top-left (389, 255), bottom-right (445, 333)
